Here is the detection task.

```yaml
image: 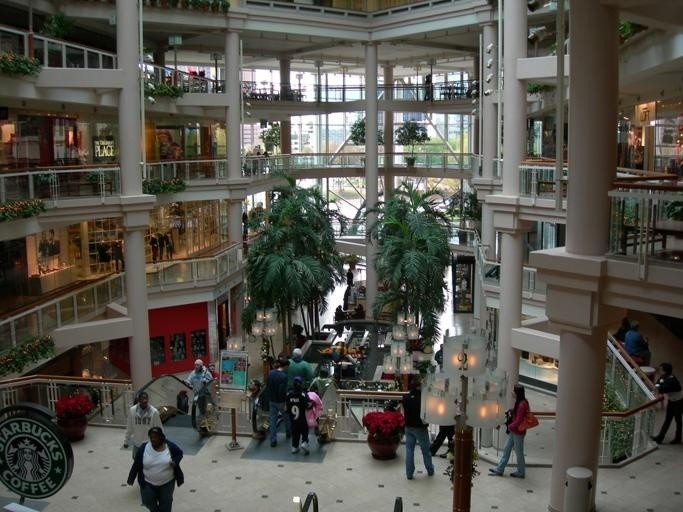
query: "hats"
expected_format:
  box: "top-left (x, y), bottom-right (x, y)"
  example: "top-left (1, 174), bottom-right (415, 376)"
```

top-left (294, 376), bottom-right (301, 382)
top-left (195, 359), bottom-right (203, 366)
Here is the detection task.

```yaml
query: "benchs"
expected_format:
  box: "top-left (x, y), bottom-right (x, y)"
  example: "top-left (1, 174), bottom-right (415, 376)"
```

top-left (621, 219), bottom-right (682, 256)
top-left (66, 179), bottom-right (112, 197)
top-left (538, 180), bottom-right (566, 197)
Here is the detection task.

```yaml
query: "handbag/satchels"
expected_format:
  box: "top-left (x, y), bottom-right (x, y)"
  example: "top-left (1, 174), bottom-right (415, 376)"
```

top-left (260, 387), bottom-right (270, 411)
top-left (518, 411), bottom-right (538, 432)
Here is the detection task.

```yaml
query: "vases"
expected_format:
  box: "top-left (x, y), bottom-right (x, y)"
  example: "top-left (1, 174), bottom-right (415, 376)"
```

top-left (363, 410), bottom-right (406, 437)
top-left (367, 433), bottom-right (400, 461)
top-left (57, 416), bottom-right (87, 442)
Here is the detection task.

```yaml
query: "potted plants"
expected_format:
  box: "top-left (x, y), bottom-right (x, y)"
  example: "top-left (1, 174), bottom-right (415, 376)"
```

top-left (393, 119), bottom-right (429, 166)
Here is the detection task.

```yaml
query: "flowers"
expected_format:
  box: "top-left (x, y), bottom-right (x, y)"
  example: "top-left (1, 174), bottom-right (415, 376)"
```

top-left (53, 392), bottom-right (93, 419)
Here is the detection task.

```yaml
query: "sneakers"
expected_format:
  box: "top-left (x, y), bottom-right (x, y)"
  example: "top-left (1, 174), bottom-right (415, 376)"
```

top-left (489, 469), bottom-right (503, 476)
top-left (510, 472), bottom-right (524, 477)
top-left (292, 445), bottom-right (300, 452)
top-left (301, 441), bottom-right (310, 454)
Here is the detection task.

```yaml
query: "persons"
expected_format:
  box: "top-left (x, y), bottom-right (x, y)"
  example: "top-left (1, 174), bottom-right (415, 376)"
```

top-left (150, 234), bottom-right (175, 261)
top-left (48, 229), bottom-right (59, 256)
top-left (123, 392), bottom-right (165, 460)
top-left (38, 232), bottom-right (49, 257)
top-left (429, 427), bottom-right (455, 458)
top-left (425, 73), bottom-right (432, 99)
top-left (99, 241), bottom-right (111, 262)
top-left (178, 267), bottom-right (371, 454)
top-left (113, 239), bottom-right (125, 273)
top-left (649, 362), bottom-right (683, 444)
top-left (489, 384), bottom-right (530, 478)
top-left (402, 380), bottom-right (434, 479)
top-left (616, 319), bottom-right (631, 343)
top-left (127, 427), bottom-right (183, 512)
top-left (242, 212), bottom-right (248, 241)
top-left (624, 320), bottom-right (652, 365)
